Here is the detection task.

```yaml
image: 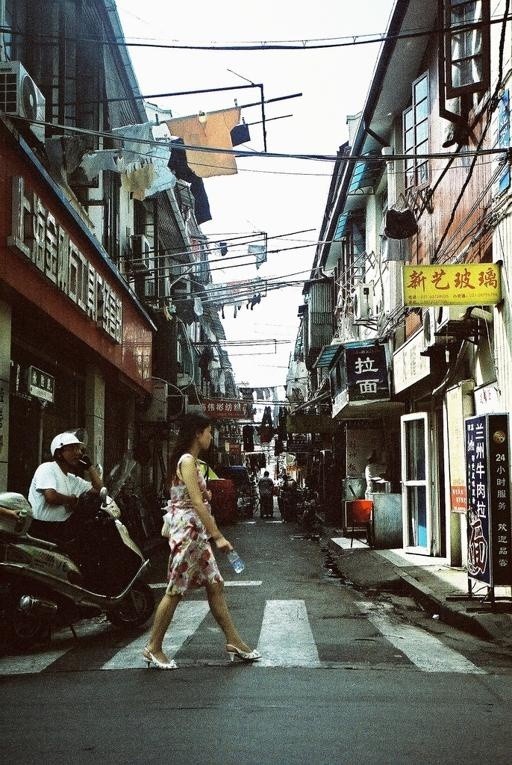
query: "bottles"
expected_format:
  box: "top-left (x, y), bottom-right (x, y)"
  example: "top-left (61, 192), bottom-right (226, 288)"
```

top-left (226, 548), bottom-right (245, 575)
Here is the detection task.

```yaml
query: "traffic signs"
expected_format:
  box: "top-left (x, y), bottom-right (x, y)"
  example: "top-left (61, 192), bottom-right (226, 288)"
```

top-left (28, 366), bottom-right (56, 404)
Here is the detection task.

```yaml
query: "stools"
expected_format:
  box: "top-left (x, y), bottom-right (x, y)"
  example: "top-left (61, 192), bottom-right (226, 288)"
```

top-left (350, 521), bottom-right (372, 549)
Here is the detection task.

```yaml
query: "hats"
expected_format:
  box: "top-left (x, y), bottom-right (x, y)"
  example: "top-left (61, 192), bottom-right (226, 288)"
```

top-left (48, 430), bottom-right (84, 457)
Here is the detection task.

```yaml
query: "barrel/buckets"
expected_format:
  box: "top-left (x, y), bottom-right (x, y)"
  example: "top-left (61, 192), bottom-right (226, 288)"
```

top-left (352, 500), bottom-right (373, 522)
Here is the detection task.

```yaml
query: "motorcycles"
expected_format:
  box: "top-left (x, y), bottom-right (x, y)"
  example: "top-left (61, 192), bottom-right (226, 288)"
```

top-left (0, 486), bottom-right (154, 655)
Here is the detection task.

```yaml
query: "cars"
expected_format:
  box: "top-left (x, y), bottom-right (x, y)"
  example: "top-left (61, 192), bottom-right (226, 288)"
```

top-left (205, 466), bottom-right (252, 521)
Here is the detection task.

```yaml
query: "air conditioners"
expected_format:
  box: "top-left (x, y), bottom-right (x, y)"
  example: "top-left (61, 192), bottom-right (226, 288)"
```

top-left (129, 235), bottom-right (150, 269)
top-left (0, 60), bottom-right (46, 144)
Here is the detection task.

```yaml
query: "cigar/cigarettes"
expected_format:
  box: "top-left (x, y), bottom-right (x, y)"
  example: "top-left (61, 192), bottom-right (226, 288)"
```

top-left (77, 459), bottom-right (87, 465)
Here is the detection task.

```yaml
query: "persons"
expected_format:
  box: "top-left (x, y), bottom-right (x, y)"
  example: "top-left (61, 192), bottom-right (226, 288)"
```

top-left (23, 432), bottom-right (120, 592)
top-left (256, 470), bottom-right (274, 519)
top-left (142, 412), bottom-right (263, 671)
top-left (319, 457), bottom-right (341, 528)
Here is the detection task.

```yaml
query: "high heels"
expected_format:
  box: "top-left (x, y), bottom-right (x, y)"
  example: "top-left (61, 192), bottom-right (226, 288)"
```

top-left (141, 647), bottom-right (180, 672)
top-left (224, 643), bottom-right (263, 663)
